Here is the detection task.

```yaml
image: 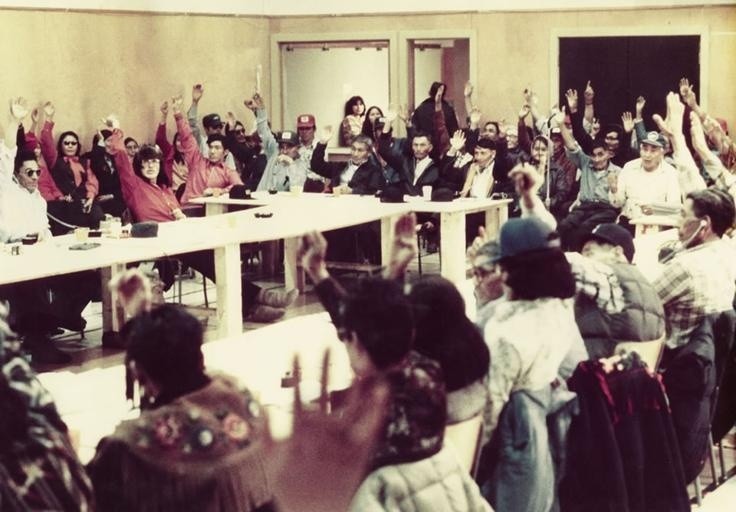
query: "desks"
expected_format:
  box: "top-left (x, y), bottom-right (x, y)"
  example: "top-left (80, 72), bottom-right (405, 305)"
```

top-left (37, 277), bottom-right (477, 464)
top-left (0, 191), bottom-right (514, 340)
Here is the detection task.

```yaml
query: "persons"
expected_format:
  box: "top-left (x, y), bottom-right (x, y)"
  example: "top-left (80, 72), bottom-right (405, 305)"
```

top-left (1, 302), bottom-right (96, 511)
top-left (650, 189), bottom-right (736, 351)
top-left (299, 229), bottom-right (446, 475)
top-left (508, 163), bottom-right (665, 359)
top-left (336, 82), bottom-right (735, 254)
top-left (466, 216), bottom-right (589, 431)
top-left (385, 213), bottom-right (491, 424)
top-left (269, 347), bottom-right (390, 511)
top-left (3, 84), bottom-right (384, 362)
top-left (83, 269), bottom-right (272, 510)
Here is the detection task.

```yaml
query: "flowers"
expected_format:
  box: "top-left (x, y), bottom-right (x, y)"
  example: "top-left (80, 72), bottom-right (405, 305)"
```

top-left (546, 113), bottom-right (556, 129)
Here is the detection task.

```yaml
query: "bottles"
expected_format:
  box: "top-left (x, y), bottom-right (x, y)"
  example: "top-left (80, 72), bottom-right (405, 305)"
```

top-left (277, 130), bottom-right (299, 145)
top-left (203, 114), bottom-right (225, 125)
top-left (578, 223), bottom-right (634, 263)
top-left (473, 218), bottom-right (561, 268)
top-left (641, 131), bottom-right (667, 148)
top-left (297, 115), bottom-right (315, 129)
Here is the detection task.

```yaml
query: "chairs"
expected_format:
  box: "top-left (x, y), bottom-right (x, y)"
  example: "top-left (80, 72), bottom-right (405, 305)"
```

top-left (254, 289), bottom-right (299, 320)
top-left (31, 347), bottom-right (73, 365)
top-left (713, 309), bottom-right (736, 492)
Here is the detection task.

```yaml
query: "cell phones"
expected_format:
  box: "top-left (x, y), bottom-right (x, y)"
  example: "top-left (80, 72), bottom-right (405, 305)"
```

top-left (378, 117), bottom-right (386, 123)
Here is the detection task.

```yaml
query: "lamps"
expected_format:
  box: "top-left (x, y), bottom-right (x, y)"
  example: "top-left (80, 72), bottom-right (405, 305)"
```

top-left (713, 309), bottom-right (736, 492)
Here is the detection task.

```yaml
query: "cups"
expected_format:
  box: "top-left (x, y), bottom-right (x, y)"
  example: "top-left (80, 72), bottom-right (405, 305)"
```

top-left (235, 128), bottom-right (245, 136)
top-left (211, 126), bottom-right (224, 129)
top-left (19, 168), bottom-right (40, 176)
top-left (62, 140), bottom-right (77, 145)
